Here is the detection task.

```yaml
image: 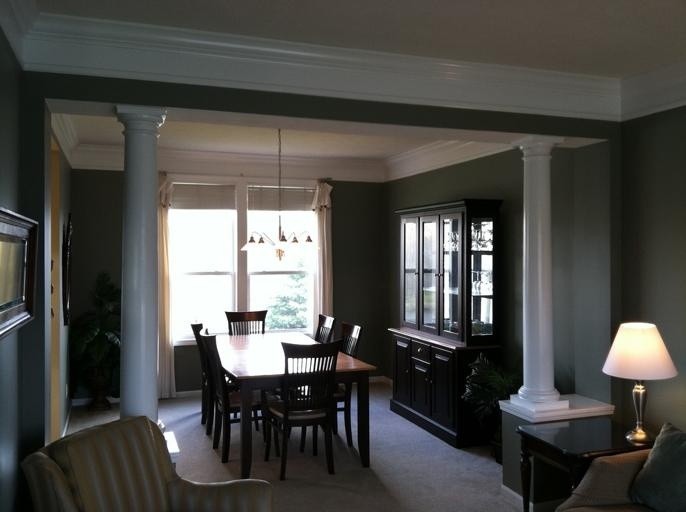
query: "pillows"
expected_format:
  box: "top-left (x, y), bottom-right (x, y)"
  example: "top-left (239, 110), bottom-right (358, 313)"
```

top-left (628, 422), bottom-right (686, 511)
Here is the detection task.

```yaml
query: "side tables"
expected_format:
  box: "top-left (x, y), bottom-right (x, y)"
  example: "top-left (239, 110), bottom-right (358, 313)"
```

top-left (516, 417), bottom-right (658, 511)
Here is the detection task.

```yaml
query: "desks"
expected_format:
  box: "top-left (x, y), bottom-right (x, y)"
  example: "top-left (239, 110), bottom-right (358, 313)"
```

top-left (207, 331), bottom-right (377, 479)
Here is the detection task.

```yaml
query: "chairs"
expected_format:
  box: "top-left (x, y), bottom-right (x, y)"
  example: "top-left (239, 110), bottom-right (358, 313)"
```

top-left (191, 310), bottom-right (363, 479)
top-left (19, 414), bottom-right (274, 512)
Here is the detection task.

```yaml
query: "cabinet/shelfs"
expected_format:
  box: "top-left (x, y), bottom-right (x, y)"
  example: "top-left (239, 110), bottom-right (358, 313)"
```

top-left (386, 193), bottom-right (512, 449)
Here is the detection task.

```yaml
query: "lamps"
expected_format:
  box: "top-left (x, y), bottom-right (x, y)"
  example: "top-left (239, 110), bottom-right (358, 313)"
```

top-left (603, 322), bottom-right (679, 448)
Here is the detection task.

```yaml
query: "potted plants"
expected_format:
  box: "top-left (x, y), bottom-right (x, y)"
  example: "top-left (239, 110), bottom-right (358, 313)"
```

top-left (68, 273), bottom-right (121, 411)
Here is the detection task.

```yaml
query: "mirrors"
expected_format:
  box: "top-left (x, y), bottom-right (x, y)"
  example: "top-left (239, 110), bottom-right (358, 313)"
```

top-left (0, 207), bottom-right (40, 338)
